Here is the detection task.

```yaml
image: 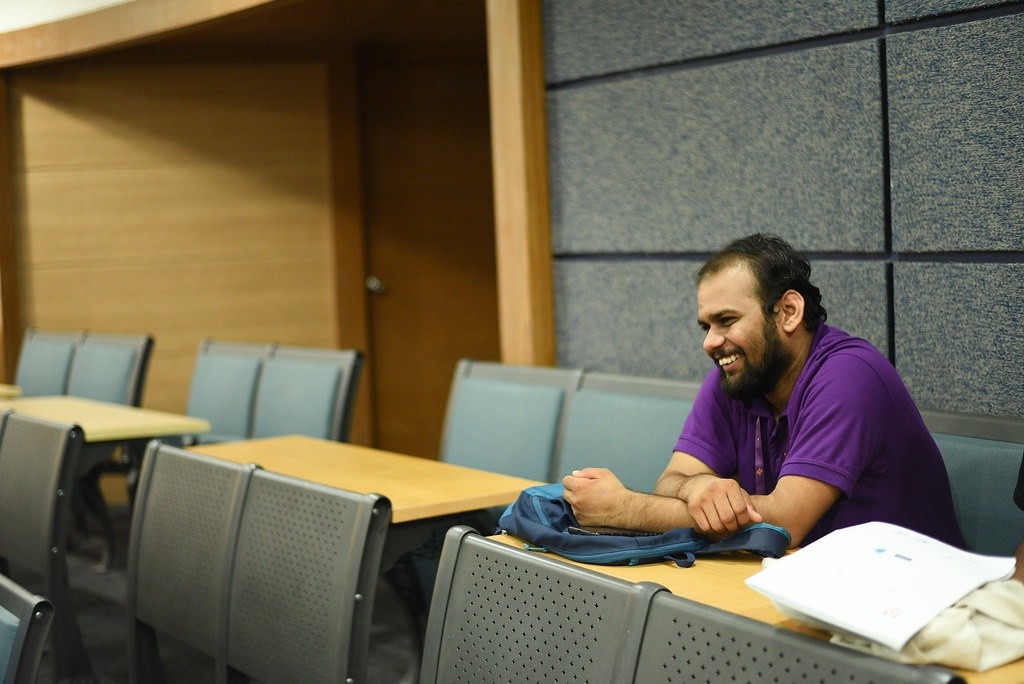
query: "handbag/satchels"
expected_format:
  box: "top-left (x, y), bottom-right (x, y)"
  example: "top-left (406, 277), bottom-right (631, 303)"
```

top-left (500, 480), bottom-right (793, 568)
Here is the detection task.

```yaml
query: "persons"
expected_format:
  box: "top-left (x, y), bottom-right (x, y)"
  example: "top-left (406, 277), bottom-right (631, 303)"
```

top-left (1010, 447), bottom-right (1024, 582)
top-left (562, 230), bottom-right (965, 551)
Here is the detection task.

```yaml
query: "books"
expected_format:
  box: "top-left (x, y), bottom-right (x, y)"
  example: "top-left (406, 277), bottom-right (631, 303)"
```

top-left (746, 522), bottom-right (1016, 652)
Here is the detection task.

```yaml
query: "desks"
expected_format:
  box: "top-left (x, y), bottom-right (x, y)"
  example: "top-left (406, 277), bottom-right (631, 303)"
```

top-left (486, 535), bottom-right (1024, 684)
top-left (0, 396), bottom-right (212, 485)
top-left (184, 432), bottom-right (557, 576)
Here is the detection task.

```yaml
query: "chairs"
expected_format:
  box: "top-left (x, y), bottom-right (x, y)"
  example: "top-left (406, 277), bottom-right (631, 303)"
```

top-left (0, 327), bottom-right (1024, 684)
